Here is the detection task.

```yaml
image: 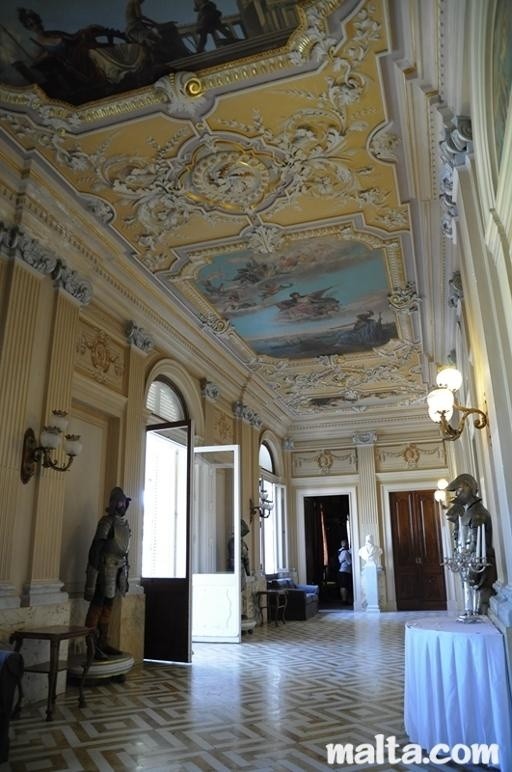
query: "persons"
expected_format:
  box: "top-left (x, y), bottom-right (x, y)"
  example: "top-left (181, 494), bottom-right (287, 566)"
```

top-left (445, 474), bottom-right (497, 616)
top-left (290, 292), bottom-right (326, 304)
top-left (17, 7), bottom-right (94, 64)
top-left (84, 487), bottom-right (133, 660)
top-left (359, 535), bottom-right (383, 568)
top-left (228, 519), bottom-right (250, 621)
top-left (337, 540), bottom-right (352, 602)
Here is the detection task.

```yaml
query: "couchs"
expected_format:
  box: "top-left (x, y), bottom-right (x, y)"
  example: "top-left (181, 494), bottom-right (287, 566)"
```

top-left (0, 649), bottom-right (24, 763)
top-left (267, 578), bottom-right (319, 621)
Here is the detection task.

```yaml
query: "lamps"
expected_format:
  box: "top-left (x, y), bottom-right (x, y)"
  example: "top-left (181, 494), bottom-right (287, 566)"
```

top-left (250, 494), bottom-right (274, 523)
top-left (426, 364), bottom-right (486, 441)
top-left (20, 410), bottom-right (83, 485)
top-left (434, 479), bottom-right (451, 509)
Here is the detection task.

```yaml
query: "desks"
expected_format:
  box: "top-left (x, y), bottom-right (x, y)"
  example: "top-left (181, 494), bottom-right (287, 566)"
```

top-left (404, 615), bottom-right (512, 772)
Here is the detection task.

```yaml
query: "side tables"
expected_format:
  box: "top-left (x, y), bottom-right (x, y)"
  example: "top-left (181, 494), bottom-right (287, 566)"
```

top-left (255, 590), bottom-right (288, 626)
top-left (8, 626), bottom-right (96, 721)
